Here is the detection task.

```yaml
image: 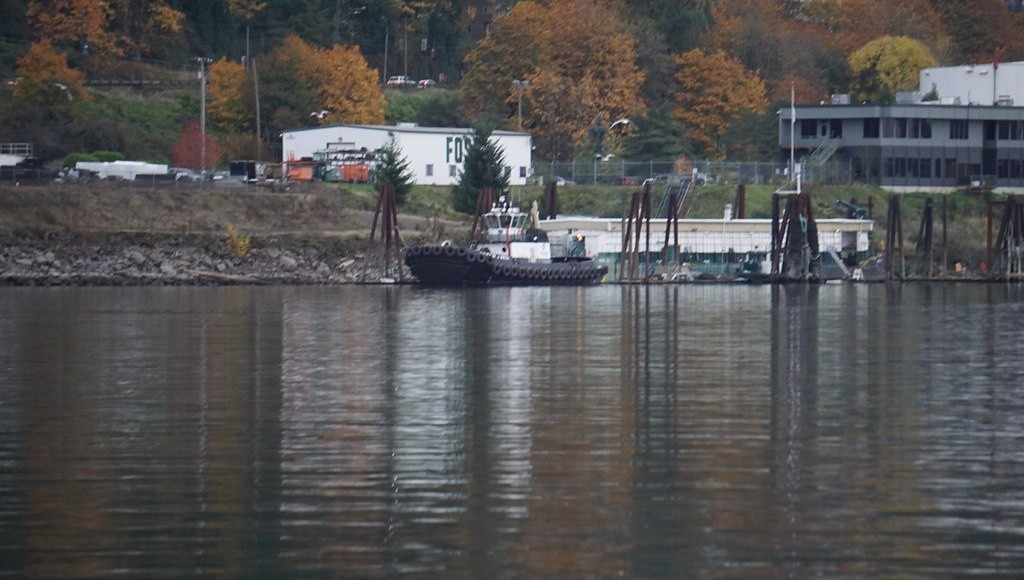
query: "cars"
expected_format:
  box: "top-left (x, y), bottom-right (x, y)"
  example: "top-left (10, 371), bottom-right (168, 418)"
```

top-left (386, 75), bottom-right (416, 84)
top-left (556, 176), bottom-right (565, 186)
top-left (418, 79), bottom-right (438, 89)
top-left (669, 173), bottom-right (705, 188)
top-left (642, 178), bottom-right (654, 186)
top-left (54, 167), bottom-right (80, 183)
top-left (650, 175), bottom-right (668, 185)
top-left (168, 168), bottom-right (192, 176)
top-left (601, 154), bottom-right (616, 162)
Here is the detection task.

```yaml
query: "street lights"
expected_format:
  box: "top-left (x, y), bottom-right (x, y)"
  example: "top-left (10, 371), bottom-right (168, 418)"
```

top-left (381, 15), bottom-right (388, 83)
top-left (195, 57), bottom-right (214, 180)
top-left (511, 80), bottom-right (530, 132)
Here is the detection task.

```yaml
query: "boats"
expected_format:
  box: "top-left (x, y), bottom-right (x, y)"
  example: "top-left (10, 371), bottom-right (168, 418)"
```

top-left (404, 194), bottom-right (608, 289)
